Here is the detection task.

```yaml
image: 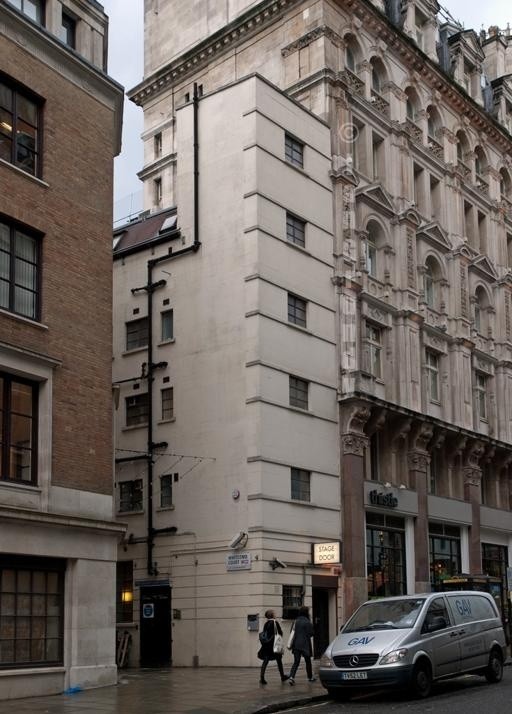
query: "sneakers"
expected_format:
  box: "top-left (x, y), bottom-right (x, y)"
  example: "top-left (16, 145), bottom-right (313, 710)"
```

top-left (281, 674), bottom-right (290, 681)
top-left (288, 678), bottom-right (296, 686)
top-left (308, 678), bottom-right (318, 681)
top-left (259, 680), bottom-right (267, 685)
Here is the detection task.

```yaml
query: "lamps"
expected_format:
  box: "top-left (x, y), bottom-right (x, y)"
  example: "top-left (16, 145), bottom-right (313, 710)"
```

top-left (122, 586), bottom-right (133, 604)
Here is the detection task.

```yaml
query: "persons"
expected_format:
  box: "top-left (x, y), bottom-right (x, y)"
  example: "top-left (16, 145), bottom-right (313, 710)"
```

top-left (260, 609), bottom-right (291, 684)
top-left (288, 606), bottom-right (317, 686)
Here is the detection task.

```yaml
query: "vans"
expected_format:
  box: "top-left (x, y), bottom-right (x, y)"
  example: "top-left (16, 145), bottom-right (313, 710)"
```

top-left (318, 590), bottom-right (506, 704)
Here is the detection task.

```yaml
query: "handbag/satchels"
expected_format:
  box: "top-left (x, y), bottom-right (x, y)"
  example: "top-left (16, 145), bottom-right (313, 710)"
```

top-left (259, 631), bottom-right (270, 646)
top-left (286, 623), bottom-right (296, 649)
top-left (273, 634), bottom-right (284, 654)
